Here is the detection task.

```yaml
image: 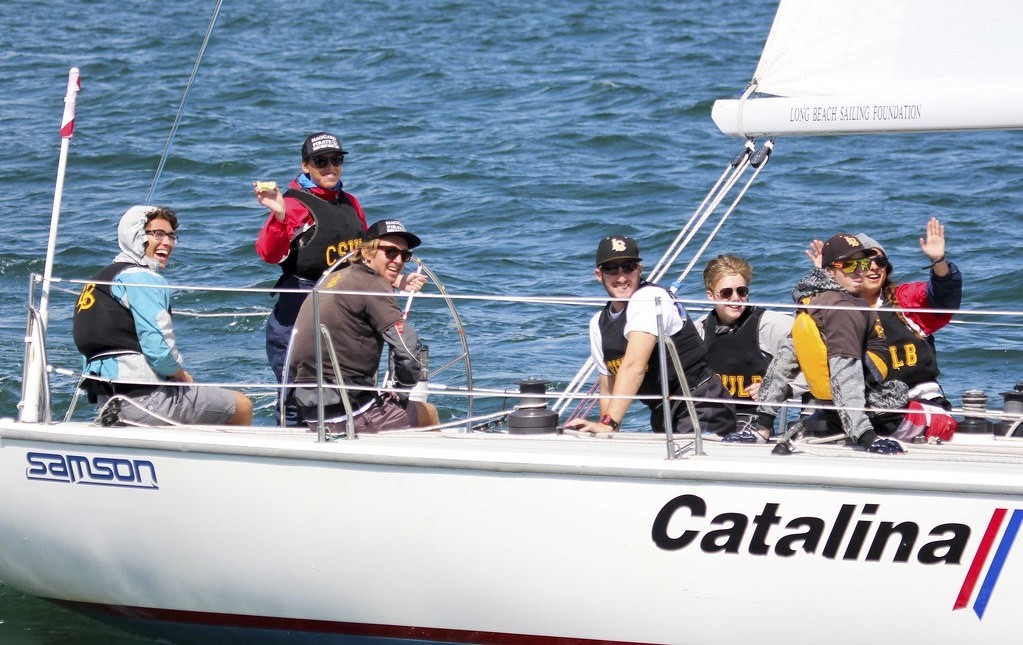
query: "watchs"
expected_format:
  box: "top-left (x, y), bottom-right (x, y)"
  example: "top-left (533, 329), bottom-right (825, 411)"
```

top-left (601, 414), bottom-right (619, 430)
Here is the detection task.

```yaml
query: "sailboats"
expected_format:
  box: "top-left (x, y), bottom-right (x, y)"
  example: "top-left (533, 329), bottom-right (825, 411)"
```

top-left (0, 0), bottom-right (1023, 645)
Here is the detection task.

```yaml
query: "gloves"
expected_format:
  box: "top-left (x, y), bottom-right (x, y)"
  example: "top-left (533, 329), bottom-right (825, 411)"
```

top-left (722, 429), bottom-right (768, 444)
top-left (866, 438), bottom-right (906, 456)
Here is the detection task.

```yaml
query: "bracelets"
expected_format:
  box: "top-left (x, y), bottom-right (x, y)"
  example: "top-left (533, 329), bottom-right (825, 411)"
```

top-left (923, 256), bottom-right (945, 271)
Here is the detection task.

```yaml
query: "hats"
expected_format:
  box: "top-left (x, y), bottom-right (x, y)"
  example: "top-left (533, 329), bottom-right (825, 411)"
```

top-left (821, 234), bottom-right (878, 268)
top-left (596, 235), bottom-right (643, 266)
top-left (301, 132), bottom-right (349, 161)
top-left (855, 233), bottom-right (889, 259)
top-left (362, 220), bottom-right (421, 249)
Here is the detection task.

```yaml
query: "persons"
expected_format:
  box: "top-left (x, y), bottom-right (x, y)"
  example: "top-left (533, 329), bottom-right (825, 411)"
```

top-left (558, 235), bottom-right (737, 436)
top-left (73, 204), bottom-right (253, 426)
top-left (254, 133), bottom-right (369, 427)
top-left (724, 217), bottom-right (962, 454)
top-left (290, 219), bottom-right (440, 434)
top-left (692, 254), bottom-right (809, 436)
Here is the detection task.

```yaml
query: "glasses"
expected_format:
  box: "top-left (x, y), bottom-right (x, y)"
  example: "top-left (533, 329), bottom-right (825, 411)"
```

top-left (829, 257), bottom-right (871, 275)
top-left (146, 229), bottom-right (179, 245)
top-left (870, 256), bottom-right (889, 268)
top-left (601, 260), bottom-right (637, 274)
top-left (711, 286), bottom-right (749, 299)
top-left (373, 246), bottom-right (412, 262)
top-left (310, 154), bottom-right (344, 168)
